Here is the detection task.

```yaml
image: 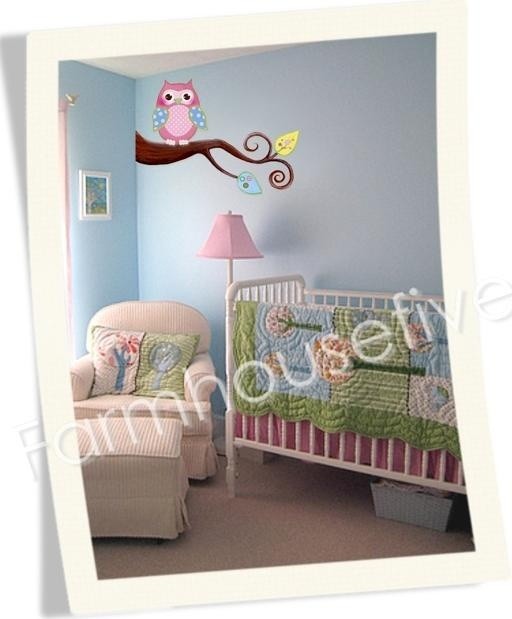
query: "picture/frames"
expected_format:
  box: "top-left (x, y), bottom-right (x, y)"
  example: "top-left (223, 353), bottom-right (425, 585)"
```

top-left (80, 169), bottom-right (113, 219)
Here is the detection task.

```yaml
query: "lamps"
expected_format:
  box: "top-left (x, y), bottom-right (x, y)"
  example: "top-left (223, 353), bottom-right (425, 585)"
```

top-left (196, 212), bottom-right (261, 298)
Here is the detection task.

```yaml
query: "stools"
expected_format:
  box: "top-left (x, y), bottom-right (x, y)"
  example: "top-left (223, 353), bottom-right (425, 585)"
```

top-left (75, 416), bottom-right (191, 540)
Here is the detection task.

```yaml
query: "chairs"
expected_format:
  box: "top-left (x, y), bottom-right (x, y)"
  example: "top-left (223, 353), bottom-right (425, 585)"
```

top-left (71, 299), bottom-right (216, 479)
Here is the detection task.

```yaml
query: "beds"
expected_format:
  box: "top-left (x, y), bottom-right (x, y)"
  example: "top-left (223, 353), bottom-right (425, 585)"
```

top-left (224, 274), bottom-right (467, 504)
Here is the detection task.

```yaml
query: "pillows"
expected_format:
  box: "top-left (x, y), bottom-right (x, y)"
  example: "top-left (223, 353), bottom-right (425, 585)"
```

top-left (85, 323), bottom-right (198, 400)
